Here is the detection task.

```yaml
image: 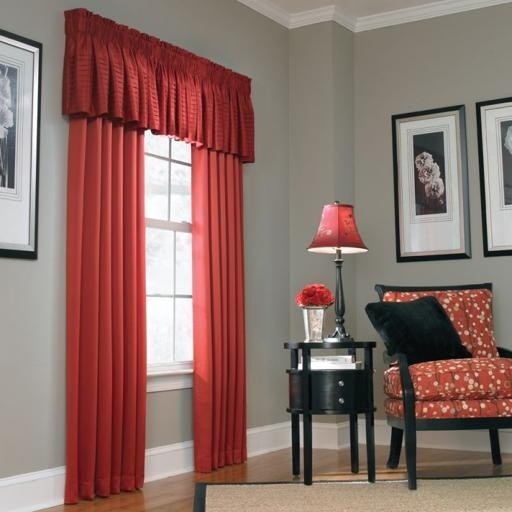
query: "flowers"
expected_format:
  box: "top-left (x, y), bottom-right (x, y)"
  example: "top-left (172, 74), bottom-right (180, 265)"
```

top-left (297, 283), bottom-right (334, 308)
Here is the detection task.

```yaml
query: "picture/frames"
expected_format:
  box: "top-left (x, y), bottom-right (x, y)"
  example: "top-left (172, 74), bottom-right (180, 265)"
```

top-left (0, 31), bottom-right (43, 260)
top-left (389, 100), bottom-right (511, 262)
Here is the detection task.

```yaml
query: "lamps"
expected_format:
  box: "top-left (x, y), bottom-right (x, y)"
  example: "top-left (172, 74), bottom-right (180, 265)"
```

top-left (306, 200), bottom-right (368, 341)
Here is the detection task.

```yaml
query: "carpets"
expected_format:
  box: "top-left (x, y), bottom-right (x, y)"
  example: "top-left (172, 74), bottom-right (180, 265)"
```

top-left (195, 474), bottom-right (511, 512)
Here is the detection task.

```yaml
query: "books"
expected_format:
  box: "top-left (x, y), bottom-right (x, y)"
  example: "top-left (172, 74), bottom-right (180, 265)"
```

top-left (300, 355), bottom-right (356, 363)
top-left (297, 361), bottom-right (362, 370)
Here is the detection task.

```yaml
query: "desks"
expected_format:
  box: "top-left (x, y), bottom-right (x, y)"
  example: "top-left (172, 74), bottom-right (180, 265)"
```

top-left (284, 341), bottom-right (377, 486)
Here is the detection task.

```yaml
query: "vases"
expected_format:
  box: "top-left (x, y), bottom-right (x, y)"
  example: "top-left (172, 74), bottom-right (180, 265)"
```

top-left (301, 307), bottom-right (325, 344)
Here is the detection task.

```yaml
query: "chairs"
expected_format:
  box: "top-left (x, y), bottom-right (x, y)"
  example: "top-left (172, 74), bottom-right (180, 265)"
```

top-left (373, 283), bottom-right (511, 489)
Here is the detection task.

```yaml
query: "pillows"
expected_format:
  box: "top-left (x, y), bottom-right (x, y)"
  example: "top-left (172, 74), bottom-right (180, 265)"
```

top-left (366, 297), bottom-right (473, 365)
